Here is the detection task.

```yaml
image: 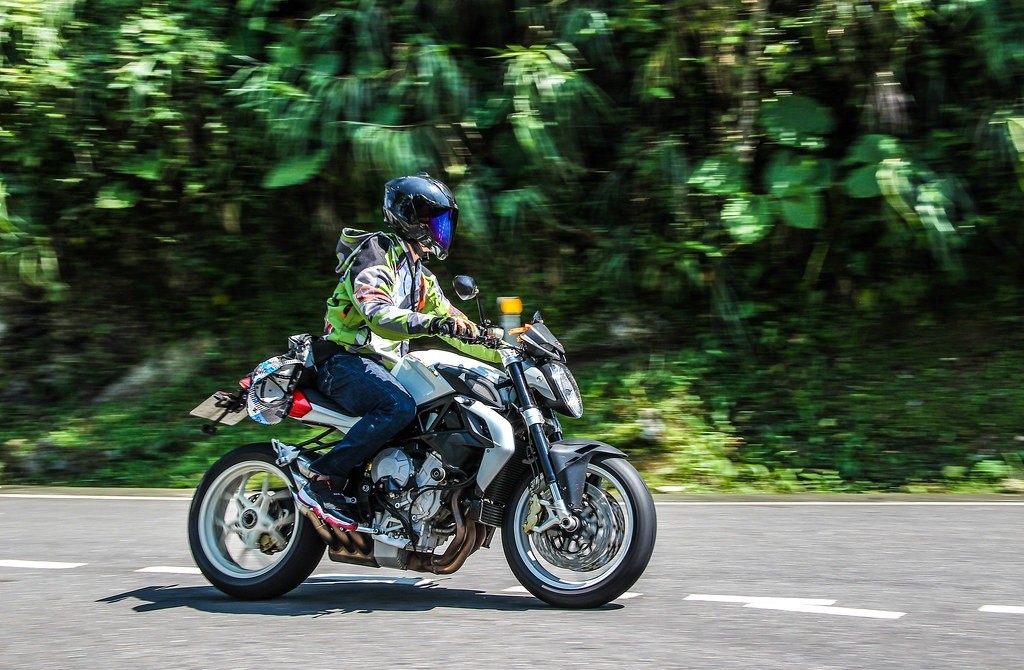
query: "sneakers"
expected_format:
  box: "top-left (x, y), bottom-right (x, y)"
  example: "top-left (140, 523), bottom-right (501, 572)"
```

top-left (296, 474), bottom-right (358, 530)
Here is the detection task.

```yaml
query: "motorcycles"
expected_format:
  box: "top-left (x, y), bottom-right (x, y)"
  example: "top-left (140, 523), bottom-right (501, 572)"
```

top-left (186, 273), bottom-right (658, 610)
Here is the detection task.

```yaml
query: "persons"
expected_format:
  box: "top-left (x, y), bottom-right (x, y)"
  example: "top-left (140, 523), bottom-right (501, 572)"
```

top-left (294, 170), bottom-right (522, 532)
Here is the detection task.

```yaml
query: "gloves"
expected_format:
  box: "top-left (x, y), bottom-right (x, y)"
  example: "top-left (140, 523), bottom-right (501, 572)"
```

top-left (431, 314), bottom-right (481, 338)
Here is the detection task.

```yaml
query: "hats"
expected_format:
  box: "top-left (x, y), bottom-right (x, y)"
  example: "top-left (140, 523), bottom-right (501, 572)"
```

top-left (246, 356), bottom-right (304, 427)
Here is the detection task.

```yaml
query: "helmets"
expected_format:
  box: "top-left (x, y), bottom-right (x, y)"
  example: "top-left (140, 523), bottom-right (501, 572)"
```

top-left (384, 171), bottom-right (459, 260)
